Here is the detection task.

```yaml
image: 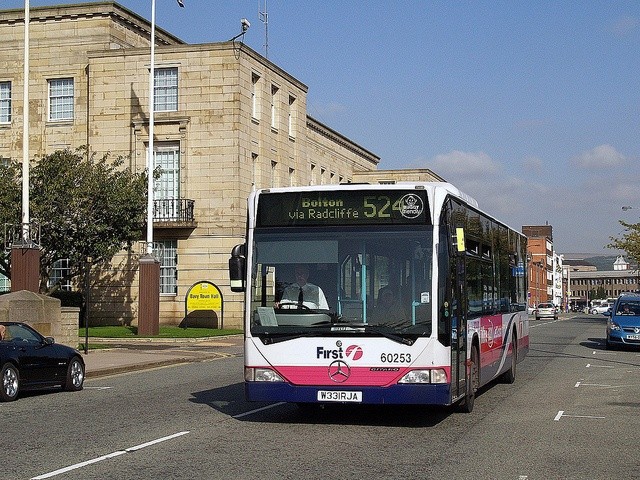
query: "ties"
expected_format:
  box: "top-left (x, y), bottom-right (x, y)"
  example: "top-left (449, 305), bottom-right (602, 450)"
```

top-left (297, 287), bottom-right (303, 309)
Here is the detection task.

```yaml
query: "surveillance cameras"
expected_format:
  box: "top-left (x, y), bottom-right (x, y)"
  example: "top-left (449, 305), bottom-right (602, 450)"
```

top-left (241, 18), bottom-right (251, 28)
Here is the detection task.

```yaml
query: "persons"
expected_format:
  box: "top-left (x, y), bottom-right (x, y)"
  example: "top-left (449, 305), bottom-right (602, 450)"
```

top-left (273, 264), bottom-right (330, 311)
top-left (621, 303), bottom-right (635, 315)
top-left (371, 273), bottom-right (423, 323)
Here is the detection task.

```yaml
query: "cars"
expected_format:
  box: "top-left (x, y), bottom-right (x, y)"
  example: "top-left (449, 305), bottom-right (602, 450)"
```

top-left (0, 322), bottom-right (86, 402)
top-left (535, 303), bottom-right (558, 320)
top-left (589, 302), bottom-right (614, 315)
top-left (603, 296), bottom-right (640, 350)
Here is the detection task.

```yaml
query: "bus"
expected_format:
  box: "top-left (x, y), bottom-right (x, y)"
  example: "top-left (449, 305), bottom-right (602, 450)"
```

top-left (228, 180), bottom-right (530, 414)
